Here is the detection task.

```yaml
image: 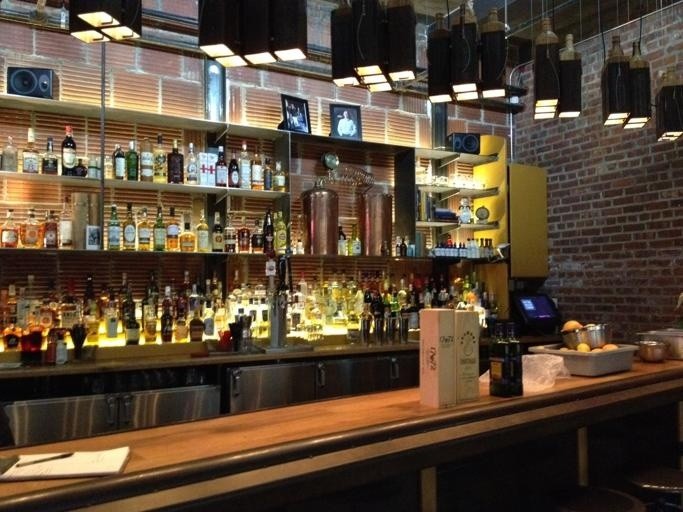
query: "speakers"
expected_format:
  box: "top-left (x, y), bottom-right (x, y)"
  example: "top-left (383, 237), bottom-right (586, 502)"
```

top-left (445, 132), bottom-right (480, 155)
top-left (6, 65), bottom-right (60, 100)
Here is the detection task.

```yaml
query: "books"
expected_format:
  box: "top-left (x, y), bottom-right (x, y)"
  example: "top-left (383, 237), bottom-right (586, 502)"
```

top-left (0, 446), bottom-right (131, 482)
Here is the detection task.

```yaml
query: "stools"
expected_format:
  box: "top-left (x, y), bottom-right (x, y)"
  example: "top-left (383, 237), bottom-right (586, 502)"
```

top-left (545, 488), bottom-right (647, 512)
top-left (624, 441), bottom-right (683, 512)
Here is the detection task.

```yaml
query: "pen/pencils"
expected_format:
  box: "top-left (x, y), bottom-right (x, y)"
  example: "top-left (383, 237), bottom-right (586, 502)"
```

top-left (16, 452), bottom-right (73, 467)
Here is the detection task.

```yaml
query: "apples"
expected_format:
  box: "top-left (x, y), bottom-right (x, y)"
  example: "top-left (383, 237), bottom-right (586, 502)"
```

top-left (602, 342), bottom-right (618, 351)
top-left (562, 318), bottom-right (581, 333)
top-left (590, 348), bottom-right (602, 353)
top-left (576, 343), bottom-right (590, 354)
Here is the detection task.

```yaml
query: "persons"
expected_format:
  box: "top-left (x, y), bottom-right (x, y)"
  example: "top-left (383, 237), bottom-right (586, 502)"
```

top-left (287, 103), bottom-right (305, 129)
top-left (337, 110), bottom-right (357, 137)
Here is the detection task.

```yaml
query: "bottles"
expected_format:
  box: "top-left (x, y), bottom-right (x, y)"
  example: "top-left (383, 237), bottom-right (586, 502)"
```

top-left (489, 322), bottom-right (522, 397)
top-left (292, 132), bottom-right (414, 257)
top-left (5, 125), bottom-right (288, 255)
top-left (414, 154), bottom-right (498, 259)
top-left (2, 262), bottom-right (499, 362)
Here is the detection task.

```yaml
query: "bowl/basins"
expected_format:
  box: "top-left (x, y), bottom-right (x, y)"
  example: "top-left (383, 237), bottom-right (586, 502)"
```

top-left (633, 341), bottom-right (671, 361)
top-left (560, 324), bottom-right (614, 349)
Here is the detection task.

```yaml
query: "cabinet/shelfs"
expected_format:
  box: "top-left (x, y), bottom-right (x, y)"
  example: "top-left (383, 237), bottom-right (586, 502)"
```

top-left (230, 358), bottom-right (393, 415)
top-left (0, 93), bottom-right (501, 350)
top-left (392, 353), bottom-right (421, 388)
top-left (3, 383), bottom-right (223, 448)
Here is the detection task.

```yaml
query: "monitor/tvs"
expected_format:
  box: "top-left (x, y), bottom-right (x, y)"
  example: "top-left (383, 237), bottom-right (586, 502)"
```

top-left (511, 290), bottom-right (561, 324)
